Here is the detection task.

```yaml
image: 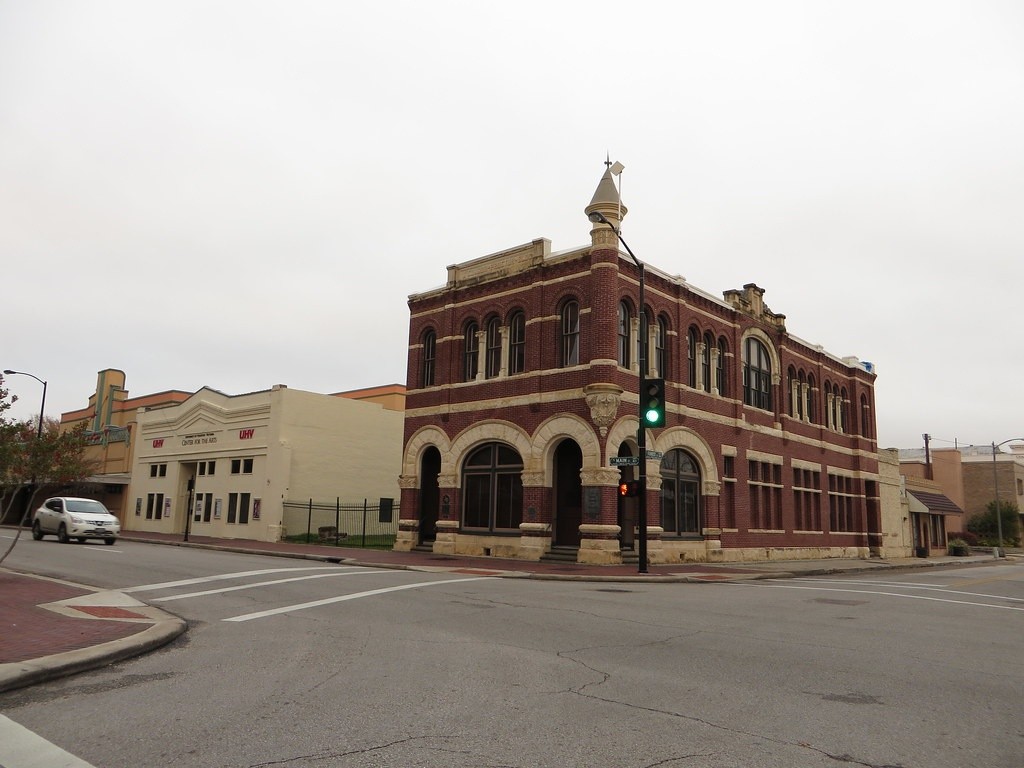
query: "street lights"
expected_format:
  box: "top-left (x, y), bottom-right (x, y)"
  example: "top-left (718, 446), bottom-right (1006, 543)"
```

top-left (3, 369), bottom-right (48, 526)
top-left (588, 211), bottom-right (650, 575)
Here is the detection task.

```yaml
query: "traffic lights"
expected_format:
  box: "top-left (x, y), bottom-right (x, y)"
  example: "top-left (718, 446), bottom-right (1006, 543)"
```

top-left (618, 479), bottom-right (638, 497)
top-left (643, 378), bottom-right (666, 429)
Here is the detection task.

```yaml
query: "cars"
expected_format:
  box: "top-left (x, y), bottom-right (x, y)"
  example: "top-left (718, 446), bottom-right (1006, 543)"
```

top-left (30, 497), bottom-right (121, 546)
